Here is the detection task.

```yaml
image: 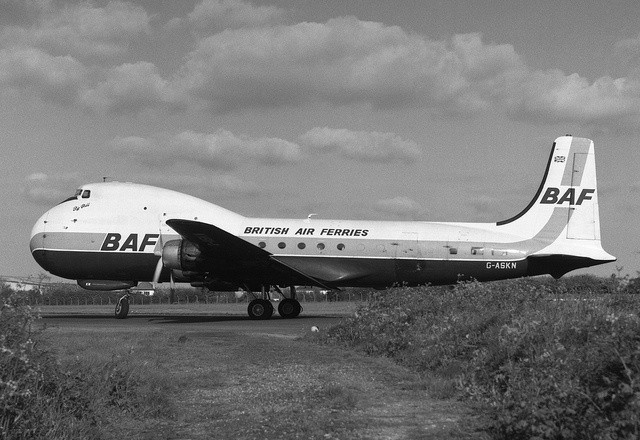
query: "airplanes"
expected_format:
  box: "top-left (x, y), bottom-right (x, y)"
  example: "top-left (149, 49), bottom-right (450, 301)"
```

top-left (29, 134), bottom-right (618, 318)
